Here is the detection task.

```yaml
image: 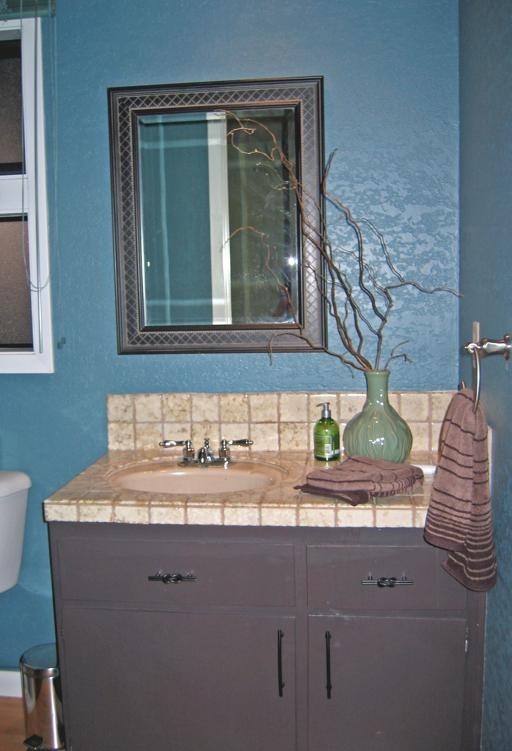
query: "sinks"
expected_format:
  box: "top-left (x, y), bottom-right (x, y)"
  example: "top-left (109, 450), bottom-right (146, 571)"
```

top-left (112, 465), bottom-right (281, 495)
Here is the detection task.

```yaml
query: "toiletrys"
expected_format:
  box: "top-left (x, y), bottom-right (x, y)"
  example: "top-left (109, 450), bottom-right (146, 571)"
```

top-left (313, 400), bottom-right (341, 462)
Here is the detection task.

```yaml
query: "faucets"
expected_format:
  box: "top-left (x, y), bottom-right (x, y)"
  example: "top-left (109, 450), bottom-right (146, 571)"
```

top-left (196, 437), bottom-right (215, 467)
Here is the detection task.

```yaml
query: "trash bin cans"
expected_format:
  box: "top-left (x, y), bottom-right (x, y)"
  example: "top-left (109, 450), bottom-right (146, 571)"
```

top-left (19, 642), bottom-right (66, 751)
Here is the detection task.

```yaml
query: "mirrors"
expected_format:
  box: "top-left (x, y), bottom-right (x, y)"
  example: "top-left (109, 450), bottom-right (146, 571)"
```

top-left (109, 77), bottom-right (329, 354)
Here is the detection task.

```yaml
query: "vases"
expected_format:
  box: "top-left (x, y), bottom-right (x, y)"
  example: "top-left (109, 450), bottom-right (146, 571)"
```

top-left (344, 370), bottom-right (411, 461)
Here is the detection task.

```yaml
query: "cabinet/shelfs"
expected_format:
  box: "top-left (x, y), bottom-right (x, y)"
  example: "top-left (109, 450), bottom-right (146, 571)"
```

top-left (48, 521), bottom-right (485, 750)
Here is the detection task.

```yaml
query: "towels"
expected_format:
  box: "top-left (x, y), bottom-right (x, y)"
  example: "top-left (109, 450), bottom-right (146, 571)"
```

top-left (294, 458), bottom-right (424, 506)
top-left (424, 389), bottom-right (498, 594)
